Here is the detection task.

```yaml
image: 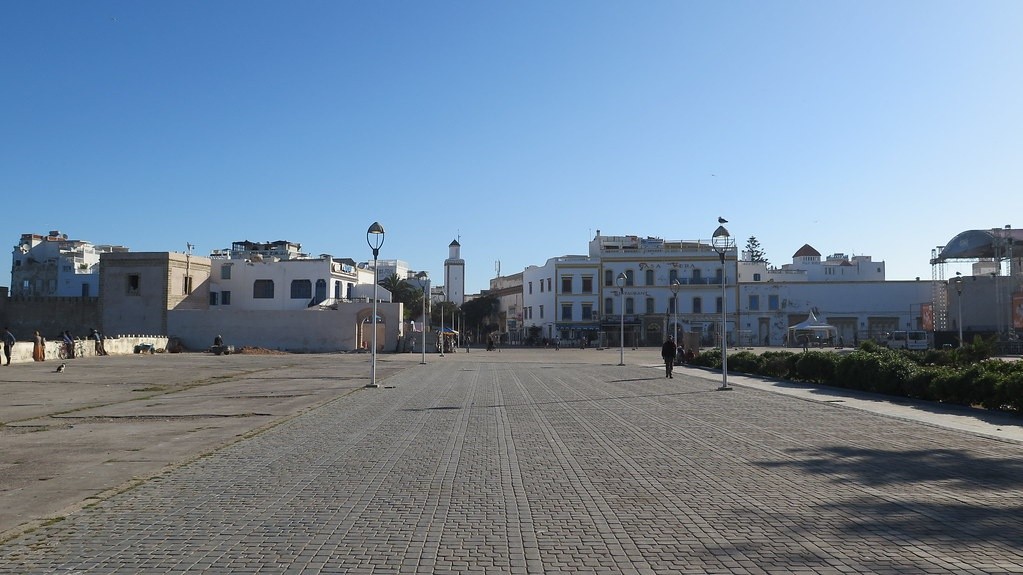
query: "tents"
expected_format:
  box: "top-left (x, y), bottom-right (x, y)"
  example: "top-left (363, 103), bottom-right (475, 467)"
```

top-left (452, 330), bottom-right (459, 347)
top-left (787, 311), bottom-right (839, 347)
top-left (436, 327), bottom-right (454, 348)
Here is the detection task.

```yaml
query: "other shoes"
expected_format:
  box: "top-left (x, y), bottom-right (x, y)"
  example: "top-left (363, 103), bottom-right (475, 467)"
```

top-left (666, 374), bottom-right (668, 377)
top-left (103, 354), bottom-right (107, 355)
top-left (3, 364), bottom-right (10, 366)
top-left (670, 377), bottom-right (673, 378)
top-left (99, 354), bottom-right (104, 356)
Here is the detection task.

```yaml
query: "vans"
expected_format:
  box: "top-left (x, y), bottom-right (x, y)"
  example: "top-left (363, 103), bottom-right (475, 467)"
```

top-left (886, 330), bottom-right (928, 350)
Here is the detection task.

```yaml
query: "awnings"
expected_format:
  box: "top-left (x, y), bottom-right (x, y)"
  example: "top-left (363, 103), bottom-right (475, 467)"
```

top-left (557, 323), bottom-right (600, 330)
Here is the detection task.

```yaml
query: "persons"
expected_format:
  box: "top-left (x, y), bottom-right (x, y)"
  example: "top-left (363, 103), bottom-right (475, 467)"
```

top-left (28, 330), bottom-right (45, 361)
top-left (89, 329), bottom-right (107, 355)
top-left (662, 335), bottom-right (676, 378)
top-left (214, 335), bottom-right (224, 345)
top-left (555, 335), bottom-right (560, 350)
top-left (803, 333), bottom-right (809, 352)
top-left (579, 337), bottom-right (587, 349)
top-left (765, 335), bottom-right (769, 346)
top-left (543, 337), bottom-right (552, 348)
top-left (466, 337), bottom-right (470, 353)
top-left (396, 332), bottom-right (400, 352)
top-left (783, 334), bottom-right (787, 347)
top-left (56, 330), bottom-right (75, 358)
top-left (1, 327), bottom-right (15, 366)
top-left (818, 337), bottom-right (823, 349)
top-left (829, 336), bottom-right (833, 346)
top-left (487, 336), bottom-right (493, 351)
top-left (408, 336), bottom-right (416, 353)
top-left (677, 346), bottom-right (697, 365)
top-left (839, 336), bottom-right (845, 349)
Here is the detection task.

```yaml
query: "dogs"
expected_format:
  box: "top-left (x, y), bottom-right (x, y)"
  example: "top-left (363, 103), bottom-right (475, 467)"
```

top-left (57, 363), bottom-right (65, 373)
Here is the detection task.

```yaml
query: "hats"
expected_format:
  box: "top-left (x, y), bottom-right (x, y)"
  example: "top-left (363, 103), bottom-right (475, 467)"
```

top-left (93, 330), bottom-right (97, 332)
top-left (218, 335), bottom-right (222, 339)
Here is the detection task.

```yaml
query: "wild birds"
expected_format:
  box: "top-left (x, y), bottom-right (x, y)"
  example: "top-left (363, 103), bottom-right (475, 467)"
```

top-left (718, 217), bottom-right (728, 226)
top-left (956, 271), bottom-right (962, 276)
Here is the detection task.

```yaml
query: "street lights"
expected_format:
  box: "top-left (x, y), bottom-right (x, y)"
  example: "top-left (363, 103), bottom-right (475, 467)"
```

top-left (616, 272), bottom-right (628, 366)
top-left (451, 301), bottom-right (467, 349)
top-left (417, 270), bottom-right (429, 364)
top-left (670, 279), bottom-right (681, 352)
top-left (366, 222), bottom-right (387, 387)
top-left (953, 276), bottom-right (964, 347)
top-left (438, 291), bottom-right (447, 357)
top-left (710, 225), bottom-right (733, 390)
